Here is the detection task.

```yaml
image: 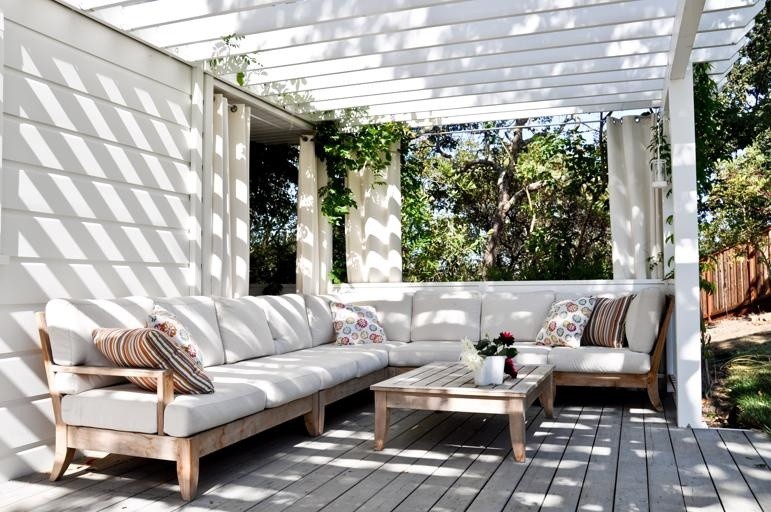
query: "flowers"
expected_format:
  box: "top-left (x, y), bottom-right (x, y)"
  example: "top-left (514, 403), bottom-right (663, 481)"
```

top-left (458, 329), bottom-right (520, 379)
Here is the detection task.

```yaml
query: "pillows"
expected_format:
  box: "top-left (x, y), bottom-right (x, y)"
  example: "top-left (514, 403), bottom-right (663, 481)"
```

top-left (328, 300), bottom-right (388, 346)
top-left (534, 293), bottom-right (638, 349)
top-left (91, 305), bottom-right (215, 395)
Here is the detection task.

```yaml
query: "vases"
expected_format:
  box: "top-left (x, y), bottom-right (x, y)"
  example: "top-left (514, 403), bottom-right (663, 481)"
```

top-left (472, 355), bottom-right (508, 386)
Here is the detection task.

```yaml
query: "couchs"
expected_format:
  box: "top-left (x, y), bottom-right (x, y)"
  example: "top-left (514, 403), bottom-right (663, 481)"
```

top-left (35, 287), bottom-right (675, 502)
